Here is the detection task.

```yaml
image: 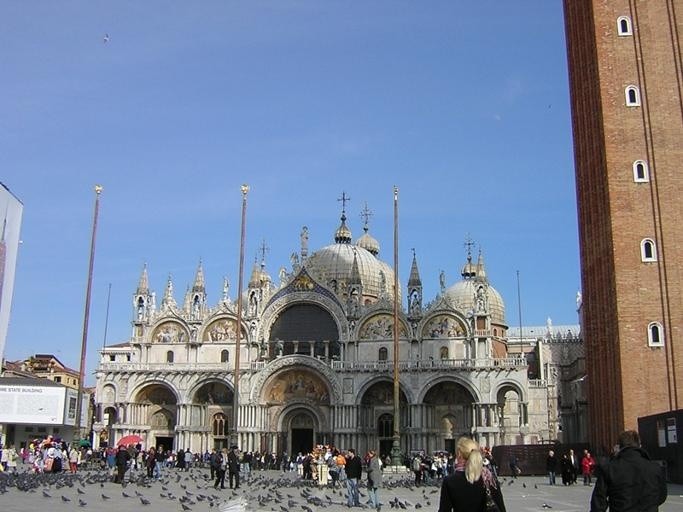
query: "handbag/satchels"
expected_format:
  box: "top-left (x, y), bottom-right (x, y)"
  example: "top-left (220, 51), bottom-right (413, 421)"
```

top-left (483, 480), bottom-right (501, 512)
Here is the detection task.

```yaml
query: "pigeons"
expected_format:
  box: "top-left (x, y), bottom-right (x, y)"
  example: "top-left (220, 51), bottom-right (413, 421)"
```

top-left (534, 483), bottom-right (538, 489)
top-left (523, 482), bottom-right (527, 488)
top-left (500, 478), bottom-right (514, 486)
top-left (542, 501), bottom-right (552, 510)
top-left (0, 469), bottom-right (444, 512)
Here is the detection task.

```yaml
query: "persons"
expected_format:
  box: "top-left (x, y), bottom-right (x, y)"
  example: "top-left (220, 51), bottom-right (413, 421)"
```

top-left (1, 432), bottom-right (194, 486)
top-left (544, 449), bottom-right (594, 486)
top-left (322, 445), bottom-right (385, 509)
top-left (485, 451), bottom-right (498, 479)
top-left (402, 450), bottom-right (455, 487)
top-left (437, 437), bottom-right (506, 512)
top-left (509, 457), bottom-right (519, 479)
top-left (588, 430), bottom-right (666, 511)
top-left (194, 445), bottom-right (314, 489)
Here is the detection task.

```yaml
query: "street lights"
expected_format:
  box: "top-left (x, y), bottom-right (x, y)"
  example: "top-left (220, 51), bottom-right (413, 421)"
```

top-left (72, 185), bottom-right (103, 448)
top-left (391, 186), bottom-right (401, 465)
top-left (229, 185), bottom-right (249, 452)
